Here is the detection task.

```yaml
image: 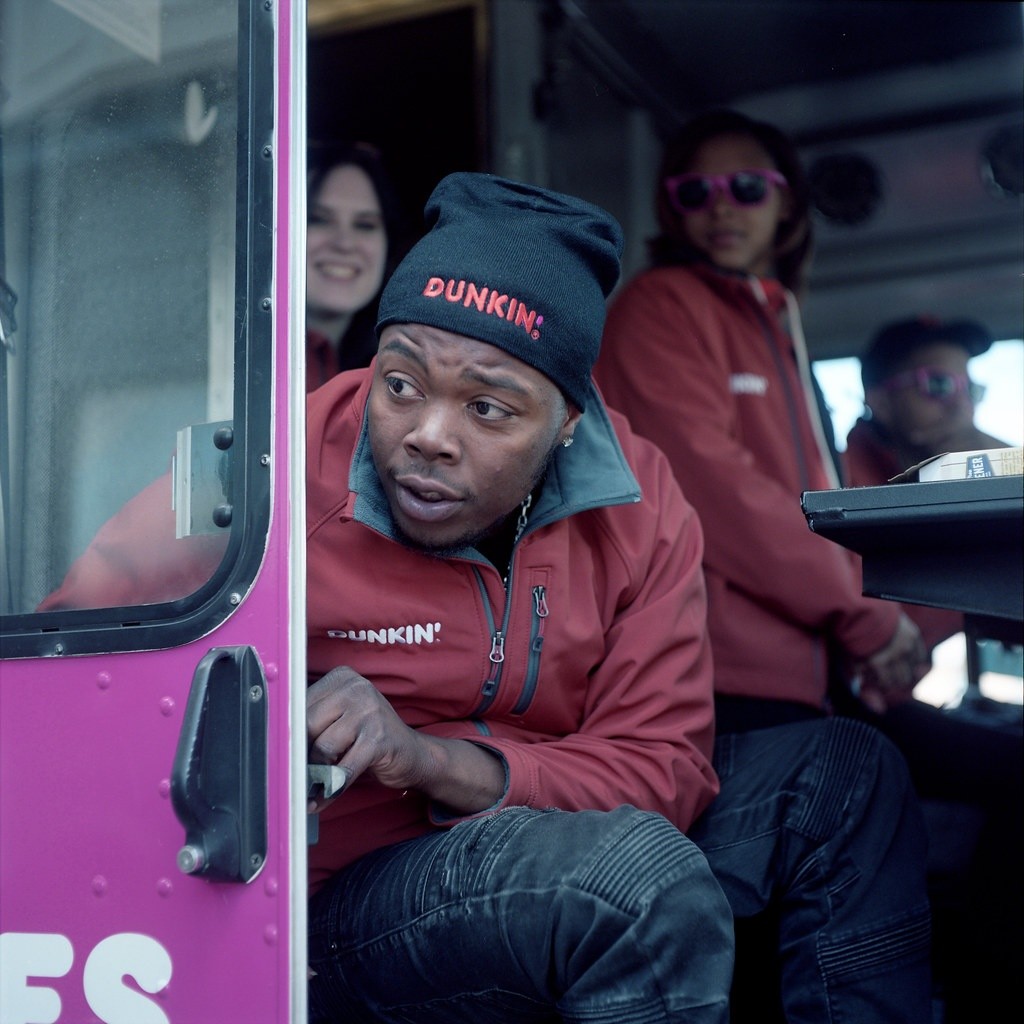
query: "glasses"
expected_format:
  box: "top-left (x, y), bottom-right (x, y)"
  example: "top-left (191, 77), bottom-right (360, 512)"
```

top-left (661, 167), bottom-right (788, 215)
top-left (884, 365), bottom-right (986, 406)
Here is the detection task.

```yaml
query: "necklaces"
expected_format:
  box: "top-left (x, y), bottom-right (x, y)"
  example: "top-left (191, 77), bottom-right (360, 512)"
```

top-left (502, 491), bottom-right (532, 591)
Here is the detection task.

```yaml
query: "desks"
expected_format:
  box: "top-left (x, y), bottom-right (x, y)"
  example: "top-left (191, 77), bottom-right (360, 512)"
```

top-left (793, 473), bottom-right (1024, 623)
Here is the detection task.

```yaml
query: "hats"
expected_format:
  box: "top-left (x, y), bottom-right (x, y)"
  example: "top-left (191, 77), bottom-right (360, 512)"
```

top-left (859, 314), bottom-right (992, 377)
top-left (374, 169), bottom-right (622, 417)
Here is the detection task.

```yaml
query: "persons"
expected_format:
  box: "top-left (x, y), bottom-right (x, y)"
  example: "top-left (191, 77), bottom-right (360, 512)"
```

top-left (305, 142), bottom-right (390, 390)
top-left (595, 115), bottom-right (938, 741)
top-left (30, 171), bottom-right (951, 1024)
top-left (845, 309), bottom-right (1024, 717)
